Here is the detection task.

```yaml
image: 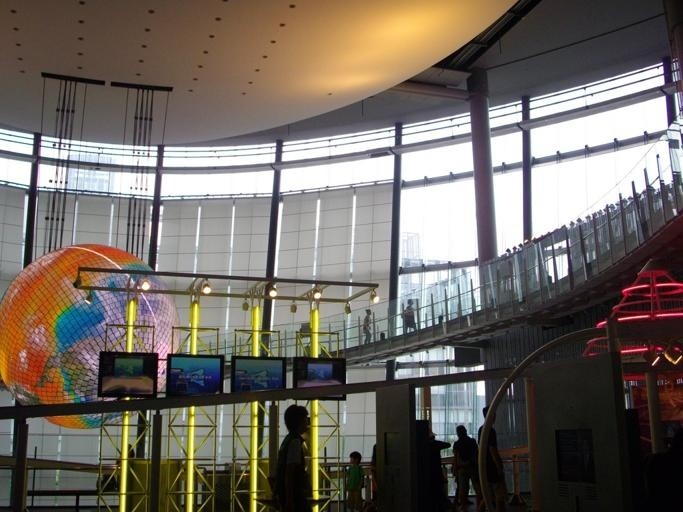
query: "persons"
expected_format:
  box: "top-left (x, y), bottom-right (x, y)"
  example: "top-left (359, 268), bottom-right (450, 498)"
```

top-left (371, 444), bottom-right (376, 502)
top-left (401, 299), bottom-right (417, 334)
top-left (346, 451), bottom-right (365, 512)
top-left (270, 404), bottom-right (311, 512)
top-left (451, 405), bottom-right (508, 511)
top-left (363, 309), bottom-right (372, 344)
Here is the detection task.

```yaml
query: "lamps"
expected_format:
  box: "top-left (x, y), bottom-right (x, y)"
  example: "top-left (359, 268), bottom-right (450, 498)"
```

top-left (84, 278), bottom-right (380, 317)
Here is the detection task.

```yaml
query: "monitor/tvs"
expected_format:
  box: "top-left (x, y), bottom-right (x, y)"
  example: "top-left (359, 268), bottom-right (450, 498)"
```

top-left (98, 351), bottom-right (158, 397)
top-left (166, 354), bottom-right (224, 397)
top-left (231, 356), bottom-right (286, 393)
top-left (293, 357), bottom-right (346, 400)
